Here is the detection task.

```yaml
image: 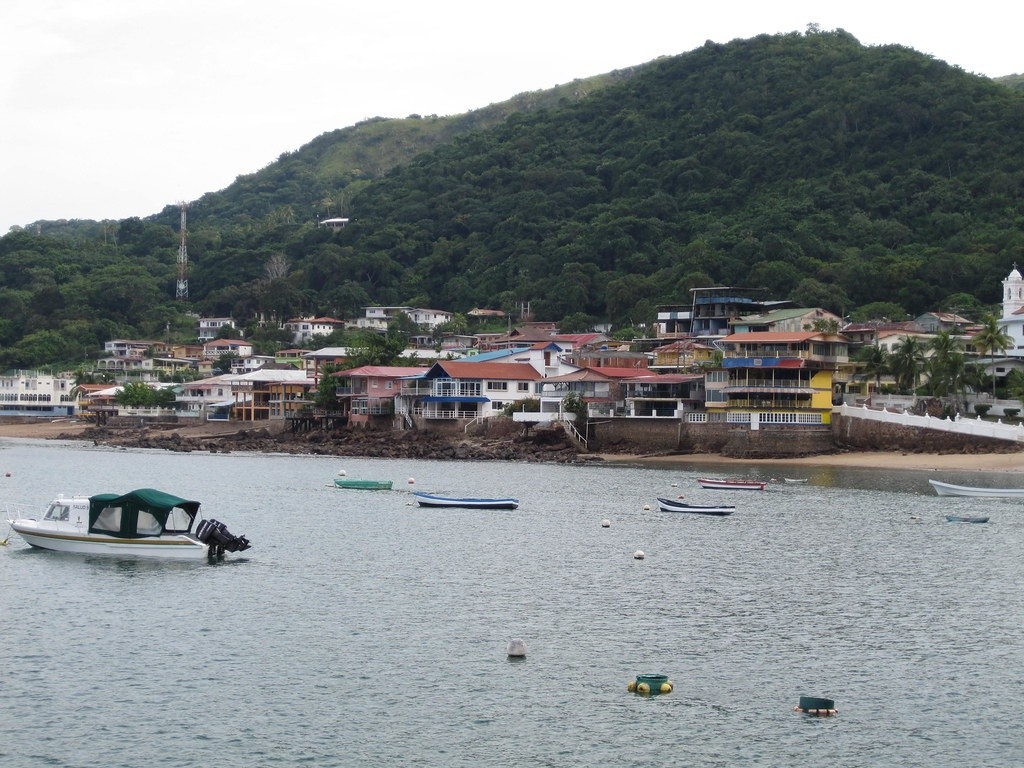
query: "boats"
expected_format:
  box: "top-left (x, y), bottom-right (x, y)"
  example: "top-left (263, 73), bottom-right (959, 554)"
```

top-left (654, 495), bottom-right (736, 519)
top-left (698, 475), bottom-right (769, 492)
top-left (409, 487), bottom-right (520, 511)
top-left (928, 477), bottom-right (1024, 499)
top-left (6, 487), bottom-right (252, 559)
top-left (331, 475), bottom-right (393, 492)
